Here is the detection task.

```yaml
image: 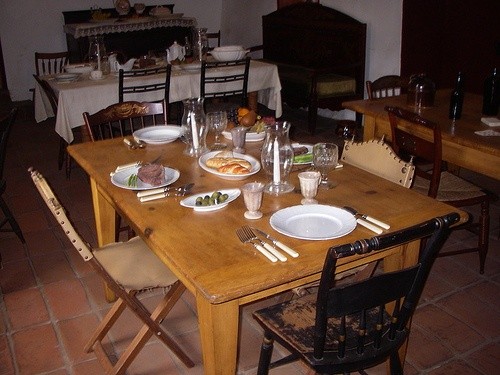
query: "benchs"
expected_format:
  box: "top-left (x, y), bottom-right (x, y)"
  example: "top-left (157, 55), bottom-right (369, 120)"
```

top-left (242, 0), bottom-right (368, 129)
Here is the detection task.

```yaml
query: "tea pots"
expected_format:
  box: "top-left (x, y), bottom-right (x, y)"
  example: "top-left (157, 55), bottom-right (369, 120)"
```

top-left (113, 0), bottom-right (130, 14)
top-left (166, 42), bottom-right (186, 64)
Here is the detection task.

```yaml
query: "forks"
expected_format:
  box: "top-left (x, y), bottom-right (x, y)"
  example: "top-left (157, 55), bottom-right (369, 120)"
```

top-left (236, 225), bottom-right (288, 262)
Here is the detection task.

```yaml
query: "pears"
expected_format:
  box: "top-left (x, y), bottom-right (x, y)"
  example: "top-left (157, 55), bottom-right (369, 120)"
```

top-left (226, 109), bottom-right (256, 131)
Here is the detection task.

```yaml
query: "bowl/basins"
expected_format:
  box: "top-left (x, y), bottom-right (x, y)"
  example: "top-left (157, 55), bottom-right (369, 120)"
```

top-left (208, 46), bottom-right (250, 61)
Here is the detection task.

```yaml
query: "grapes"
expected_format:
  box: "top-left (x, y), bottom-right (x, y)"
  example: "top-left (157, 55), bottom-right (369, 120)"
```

top-left (226, 105), bottom-right (239, 124)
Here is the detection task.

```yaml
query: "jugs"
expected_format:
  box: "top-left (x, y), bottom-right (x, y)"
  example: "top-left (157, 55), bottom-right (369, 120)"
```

top-left (407, 73), bottom-right (436, 109)
top-left (107, 54), bottom-right (118, 73)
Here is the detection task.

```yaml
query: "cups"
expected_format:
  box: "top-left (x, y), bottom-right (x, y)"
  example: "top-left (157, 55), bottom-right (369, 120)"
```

top-left (114, 59), bottom-right (136, 79)
top-left (91, 62), bottom-right (109, 79)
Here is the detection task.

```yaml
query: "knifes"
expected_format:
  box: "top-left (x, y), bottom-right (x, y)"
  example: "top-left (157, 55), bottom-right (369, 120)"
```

top-left (252, 228), bottom-right (299, 258)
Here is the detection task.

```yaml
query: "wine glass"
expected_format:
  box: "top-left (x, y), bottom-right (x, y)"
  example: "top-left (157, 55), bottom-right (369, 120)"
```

top-left (242, 182), bottom-right (264, 219)
top-left (207, 112), bottom-right (249, 153)
top-left (298, 143), bottom-right (338, 204)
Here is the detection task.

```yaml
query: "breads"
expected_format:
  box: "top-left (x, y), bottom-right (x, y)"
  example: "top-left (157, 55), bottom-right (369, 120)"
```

top-left (206, 156), bottom-right (252, 174)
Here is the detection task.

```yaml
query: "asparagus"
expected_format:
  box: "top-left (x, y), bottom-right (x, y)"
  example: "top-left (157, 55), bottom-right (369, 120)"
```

top-left (127, 174), bottom-right (138, 188)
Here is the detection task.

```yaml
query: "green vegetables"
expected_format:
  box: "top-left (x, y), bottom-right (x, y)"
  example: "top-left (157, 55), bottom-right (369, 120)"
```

top-left (292, 153), bottom-right (314, 164)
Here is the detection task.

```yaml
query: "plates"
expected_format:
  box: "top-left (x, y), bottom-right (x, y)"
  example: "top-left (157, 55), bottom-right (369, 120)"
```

top-left (265, 145), bottom-right (313, 164)
top-left (221, 131), bottom-right (266, 141)
top-left (199, 151), bottom-right (261, 180)
top-left (180, 189), bottom-right (241, 212)
top-left (54, 64), bottom-right (96, 81)
top-left (270, 204), bottom-right (358, 240)
top-left (111, 167), bottom-right (180, 190)
top-left (89, 77), bottom-right (104, 80)
top-left (182, 65), bottom-right (202, 71)
top-left (133, 125), bottom-right (184, 144)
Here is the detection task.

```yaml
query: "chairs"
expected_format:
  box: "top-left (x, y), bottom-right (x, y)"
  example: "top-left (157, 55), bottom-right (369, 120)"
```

top-left (339, 135), bottom-right (419, 193)
top-left (27, 166), bottom-right (200, 375)
top-left (179, 56), bottom-right (254, 123)
top-left (106, 61), bottom-right (174, 137)
top-left (383, 103), bottom-right (496, 279)
top-left (29, 51), bottom-right (73, 124)
top-left (251, 207), bottom-right (461, 375)
top-left (203, 27), bottom-right (222, 50)
top-left (363, 74), bottom-right (412, 100)
top-left (31, 74), bottom-right (74, 177)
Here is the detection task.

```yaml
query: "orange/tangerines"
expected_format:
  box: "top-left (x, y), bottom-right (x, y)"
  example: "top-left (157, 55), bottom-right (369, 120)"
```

top-left (237, 108), bottom-right (249, 116)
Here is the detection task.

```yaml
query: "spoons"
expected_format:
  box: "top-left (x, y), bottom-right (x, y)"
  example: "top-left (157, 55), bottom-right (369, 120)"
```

top-left (137, 183), bottom-right (194, 202)
top-left (344, 207), bottom-right (390, 234)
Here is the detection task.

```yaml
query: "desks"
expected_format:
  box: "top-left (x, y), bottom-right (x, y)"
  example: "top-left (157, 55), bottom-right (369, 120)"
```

top-left (33, 49), bottom-right (283, 180)
top-left (338, 93), bottom-right (500, 186)
top-left (62, 15), bottom-right (199, 67)
top-left (66, 122), bottom-right (469, 375)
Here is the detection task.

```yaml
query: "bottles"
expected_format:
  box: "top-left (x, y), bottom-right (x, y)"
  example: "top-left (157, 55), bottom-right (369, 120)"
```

top-left (483, 67), bottom-right (500, 117)
top-left (448, 73), bottom-right (464, 120)
top-left (185, 38), bottom-right (193, 63)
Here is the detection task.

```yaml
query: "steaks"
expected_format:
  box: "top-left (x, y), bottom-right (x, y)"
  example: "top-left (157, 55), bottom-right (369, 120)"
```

top-left (279, 146), bottom-right (308, 160)
top-left (138, 164), bottom-right (164, 187)
